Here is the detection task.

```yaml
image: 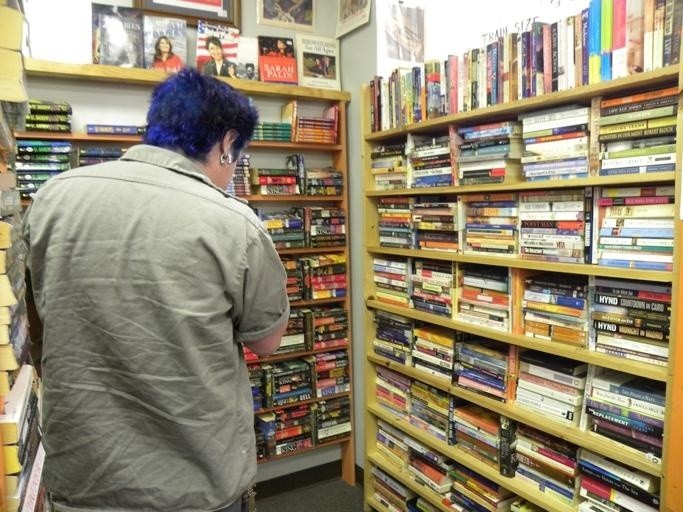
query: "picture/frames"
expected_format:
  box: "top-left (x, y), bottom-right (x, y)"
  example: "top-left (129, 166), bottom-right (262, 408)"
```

top-left (255, 1), bottom-right (316, 35)
top-left (133, 0), bottom-right (242, 36)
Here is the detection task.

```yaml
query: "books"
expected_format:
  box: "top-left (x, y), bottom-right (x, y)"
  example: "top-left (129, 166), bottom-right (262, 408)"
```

top-left (91, 0), bottom-right (341, 91)
top-left (226, 106), bottom-right (344, 196)
top-left (378, 185), bottom-right (674, 271)
top-left (281, 252), bottom-right (348, 301)
top-left (7, 98), bottom-right (147, 200)
top-left (371, 310), bottom-right (666, 511)
top-left (254, 395), bottom-right (351, 460)
top-left (254, 203), bottom-right (347, 249)
top-left (242, 307), bottom-right (352, 410)
top-left (370, 1), bottom-right (683, 133)
top-left (370, 85), bottom-right (678, 190)
top-left (372, 259), bottom-right (672, 367)
top-left (1, 1), bottom-right (51, 511)
